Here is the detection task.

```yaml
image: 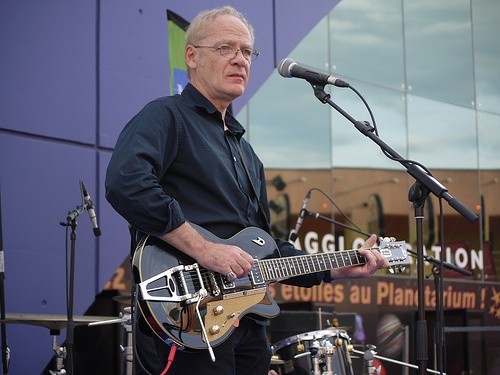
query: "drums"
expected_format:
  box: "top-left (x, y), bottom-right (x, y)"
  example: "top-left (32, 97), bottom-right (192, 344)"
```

top-left (271, 330), bottom-right (354, 375)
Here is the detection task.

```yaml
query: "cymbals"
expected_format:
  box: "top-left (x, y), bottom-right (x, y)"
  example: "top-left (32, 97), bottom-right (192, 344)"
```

top-left (6, 313), bottom-right (122, 330)
top-left (113, 294), bottom-right (135, 304)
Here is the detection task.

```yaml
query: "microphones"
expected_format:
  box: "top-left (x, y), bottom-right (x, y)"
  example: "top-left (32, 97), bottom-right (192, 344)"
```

top-left (81, 181), bottom-right (101, 236)
top-left (277, 58), bottom-right (348, 87)
top-left (290, 191), bottom-right (311, 241)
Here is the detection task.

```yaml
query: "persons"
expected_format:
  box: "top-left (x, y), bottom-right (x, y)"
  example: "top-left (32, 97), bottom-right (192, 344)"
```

top-left (105, 6), bottom-right (387, 375)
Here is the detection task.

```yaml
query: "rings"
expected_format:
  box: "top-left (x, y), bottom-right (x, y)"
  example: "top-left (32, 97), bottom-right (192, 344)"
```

top-left (226, 272), bottom-right (235, 279)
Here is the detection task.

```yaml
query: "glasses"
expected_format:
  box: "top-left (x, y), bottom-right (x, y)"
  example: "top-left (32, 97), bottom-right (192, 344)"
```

top-left (193, 45), bottom-right (260, 61)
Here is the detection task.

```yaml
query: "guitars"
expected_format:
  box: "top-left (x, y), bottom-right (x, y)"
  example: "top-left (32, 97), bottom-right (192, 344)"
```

top-left (131, 221), bottom-right (409, 362)
